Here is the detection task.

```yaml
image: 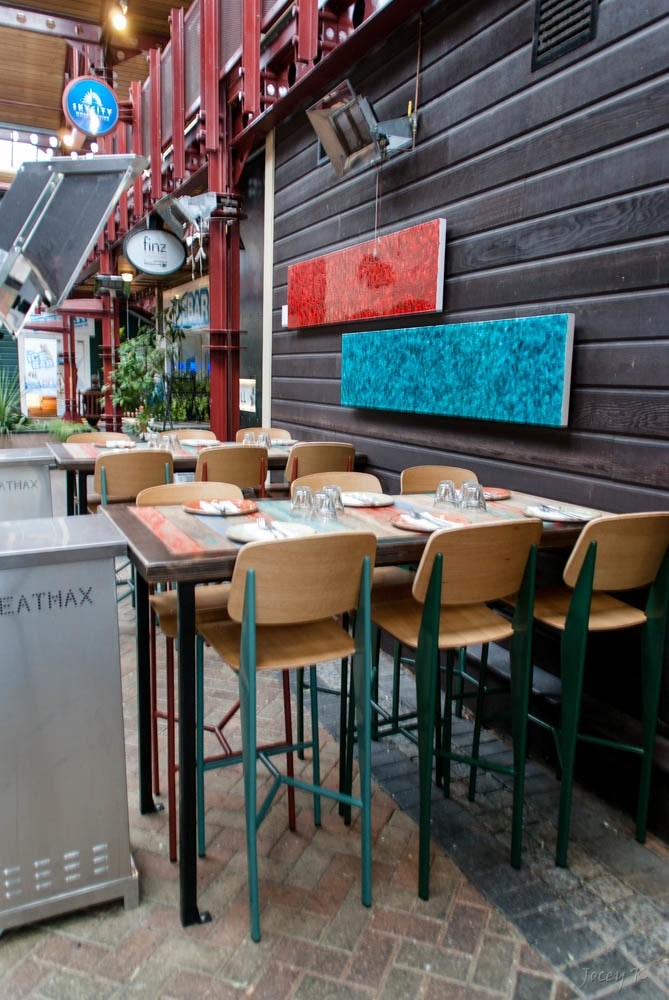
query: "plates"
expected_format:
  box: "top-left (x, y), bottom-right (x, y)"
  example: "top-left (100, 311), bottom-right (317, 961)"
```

top-left (393, 515), bottom-right (472, 534)
top-left (95, 440), bottom-right (300, 452)
top-left (523, 505), bottom-right (602, 522)
top-left (455, 486), bottom-right (511, 500)
top-left (342, 491), bottom-right (394, 507)
top-left (184, 498), bottom-right (257, 516)
top-left (226, 521), bottom-right (317, 543)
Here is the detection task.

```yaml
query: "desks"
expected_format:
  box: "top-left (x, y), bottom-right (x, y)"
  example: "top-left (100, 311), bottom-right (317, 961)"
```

top-left (45, 439), bottom-right (368, 515)
top-left (97, 486), bottom-right (623, 928)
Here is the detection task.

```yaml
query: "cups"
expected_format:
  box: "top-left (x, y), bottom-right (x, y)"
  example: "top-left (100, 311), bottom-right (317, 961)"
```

top-left (148, 432), bottom-right (183, 454)
top-left (27, 407), bottom-right (41, 416)
top-left (290, 484), bottom-right (344, 522)
top-left (242, 431), bottom-right (273, 449)
top-left (436, 478), bottom-right (488, 514)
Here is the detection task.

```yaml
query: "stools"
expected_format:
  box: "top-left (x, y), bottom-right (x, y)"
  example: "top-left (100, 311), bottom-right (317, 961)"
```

top-left (67, 428), bottom-right (669, 945)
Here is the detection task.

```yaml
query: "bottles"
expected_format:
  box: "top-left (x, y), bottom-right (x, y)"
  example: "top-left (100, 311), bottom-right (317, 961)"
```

top-left (41, 396), bottom-right (56, 417)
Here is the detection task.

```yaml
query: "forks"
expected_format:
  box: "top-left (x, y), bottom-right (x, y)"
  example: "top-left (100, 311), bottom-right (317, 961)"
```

top-left (258, 518), bottom-right (277, 538)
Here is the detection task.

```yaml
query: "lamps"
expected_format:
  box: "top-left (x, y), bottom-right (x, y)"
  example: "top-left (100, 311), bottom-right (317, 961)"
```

top-left (120, 270), bottom-right (133, 282)
top-left (152, 191), bottom-right (218, 243)
top-left (305, 78), bottom-right (414, 180)
